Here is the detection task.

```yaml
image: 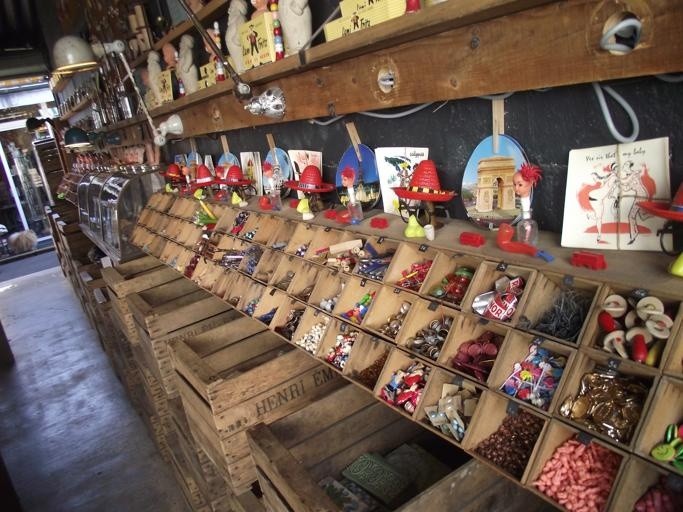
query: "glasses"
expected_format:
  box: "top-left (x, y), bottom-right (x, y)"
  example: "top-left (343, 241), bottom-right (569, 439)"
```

top-left (398, 201), bottom-right (450, 228)
top-left (656, 221), bottom-right (683, 257)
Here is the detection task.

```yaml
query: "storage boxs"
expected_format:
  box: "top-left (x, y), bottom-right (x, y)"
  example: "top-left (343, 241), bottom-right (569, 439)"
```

top-left (166, 317), bottom-right (343, 498)
top-left (43, 200), bottom-right (266, 461)
top-left (246, 385), bottom-right (540, 511)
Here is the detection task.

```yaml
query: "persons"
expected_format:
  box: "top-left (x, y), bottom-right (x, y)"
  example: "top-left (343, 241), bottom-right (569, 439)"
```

top-left (142, 0), bottom-right (313, 105)
top-left (342, 165), bottom-right (353, 206)
top-left (262, 160), bottom-right (277, 196)
top-left (509, 162), bottom-right (543, 242)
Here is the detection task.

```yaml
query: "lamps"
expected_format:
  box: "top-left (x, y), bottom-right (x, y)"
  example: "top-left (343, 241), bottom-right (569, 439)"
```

top-left (50, 32), bottom-right (186, 148)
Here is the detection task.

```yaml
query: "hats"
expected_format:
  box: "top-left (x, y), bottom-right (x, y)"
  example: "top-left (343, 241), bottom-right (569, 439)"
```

top-left (159, 164), bottom-right (184, 180)
top-left (391, 159), bottom-right (456, 202)
top-left (217, 165), bottom-right (253, 186)
top-left (283, 165), bottom-right (336, 193)
top-left (636, 183), bottom-right (683, 221)
top-left (189, 164), bottom-right (218, 186)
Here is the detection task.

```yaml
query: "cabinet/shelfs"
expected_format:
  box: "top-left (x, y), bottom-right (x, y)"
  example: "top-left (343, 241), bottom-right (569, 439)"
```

top-left (74, 163), bottom-right (168, 264)
top-left (134, 189), bottom-right (680, 511)
